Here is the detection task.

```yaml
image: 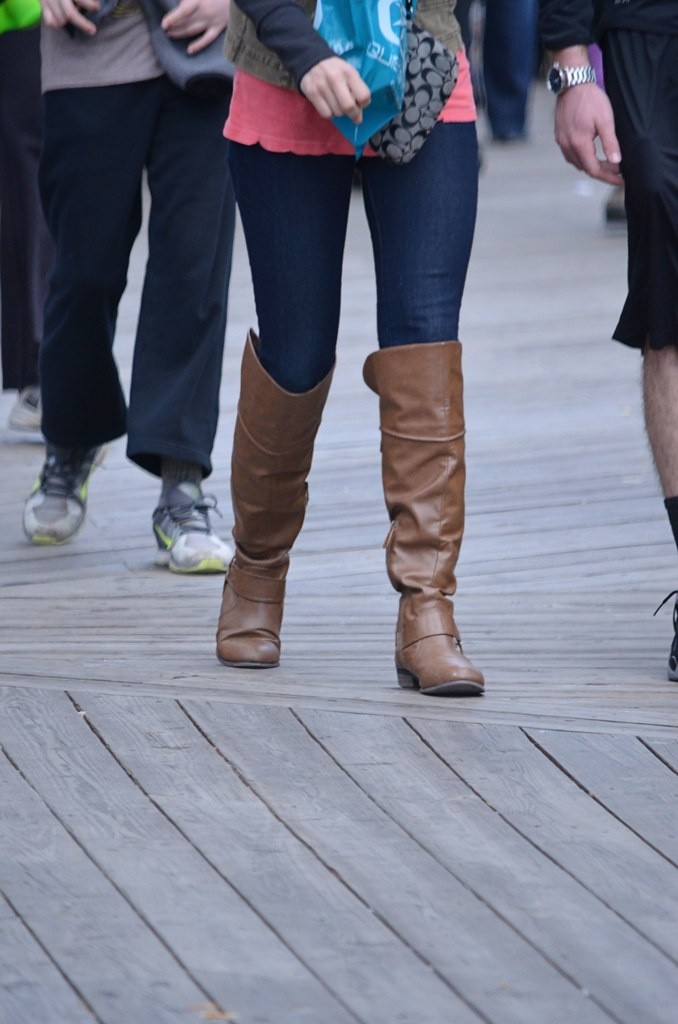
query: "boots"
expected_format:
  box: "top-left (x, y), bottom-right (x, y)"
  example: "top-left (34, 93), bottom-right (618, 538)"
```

top-left (363, 337), bottom-right (485, 697)
top-left (215, 330), bottom-right (337, 668)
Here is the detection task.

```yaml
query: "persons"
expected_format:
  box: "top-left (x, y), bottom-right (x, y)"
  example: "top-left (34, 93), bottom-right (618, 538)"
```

top-left (215, 1), bottom-right (485, 696)
top-left (535, 0), bottom-right (678, 684)
top-left (457, 0), bottom-right (532, 144)
top-left (0, 0), bottom-right (236, 575)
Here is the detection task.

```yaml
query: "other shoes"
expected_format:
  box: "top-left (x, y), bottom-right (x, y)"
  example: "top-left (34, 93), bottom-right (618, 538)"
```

top-left (604, 181), bottom-right (627, 221)
top-left (652, 589), bottom-right (678, 683)
top-left (7, 382), bottom-right (44, 430)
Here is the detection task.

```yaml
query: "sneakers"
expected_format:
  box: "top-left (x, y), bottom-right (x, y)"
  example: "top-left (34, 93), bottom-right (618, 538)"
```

top-left (23, 434), bottom-right (107, 544)
top-left (152, 498), bottom-right (234, 574)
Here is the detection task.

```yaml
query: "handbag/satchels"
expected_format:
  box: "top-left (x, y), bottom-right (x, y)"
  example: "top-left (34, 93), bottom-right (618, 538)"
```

top-left (304, 0), bottom-right (458, 164)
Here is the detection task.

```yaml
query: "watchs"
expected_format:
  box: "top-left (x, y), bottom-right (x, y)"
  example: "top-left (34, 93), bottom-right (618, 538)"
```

top-left (543, 61), bottom-right (599, 97)
